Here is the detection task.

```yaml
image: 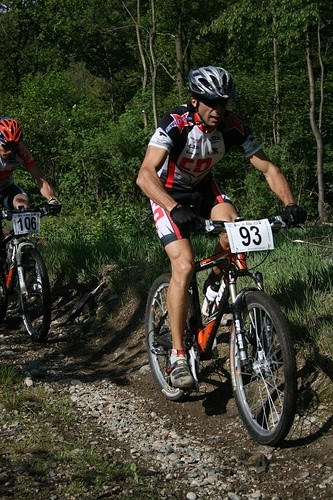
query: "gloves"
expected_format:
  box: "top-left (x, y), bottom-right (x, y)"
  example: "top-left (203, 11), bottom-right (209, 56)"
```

top-left (284, 205), bottom-right (307, 225)
top-left (170, 203), bottom-right (202, 238)
top-left (48, 198), bottom-right (62, 213)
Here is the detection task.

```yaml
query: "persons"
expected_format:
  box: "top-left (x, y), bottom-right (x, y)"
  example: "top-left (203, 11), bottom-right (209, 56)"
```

top-left (0, 118), bottom-right (62, 303)
top-left (136, 64), bottom-right (308, 390)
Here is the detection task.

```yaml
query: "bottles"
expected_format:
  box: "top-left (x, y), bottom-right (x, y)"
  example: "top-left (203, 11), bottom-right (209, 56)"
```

top-left (201, 282), bottom-right (221, 317)
top-left (6, 242), bottom-right (13, 263)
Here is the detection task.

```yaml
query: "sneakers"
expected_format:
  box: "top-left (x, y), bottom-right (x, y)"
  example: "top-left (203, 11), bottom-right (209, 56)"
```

top-left (33, 283), bottom-right (53, 303)
top-left (202, 296), bottom-right (234, 325)
top-left (0, 287), bottom-right (7, 297)
top-left (168, 358), bottom-right (195, 388)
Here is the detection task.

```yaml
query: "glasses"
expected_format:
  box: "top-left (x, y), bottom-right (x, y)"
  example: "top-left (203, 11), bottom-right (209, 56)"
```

top-left (201, 99), bottom-right (228, 107)
top-left (1, 145), bottom-right (18, 151)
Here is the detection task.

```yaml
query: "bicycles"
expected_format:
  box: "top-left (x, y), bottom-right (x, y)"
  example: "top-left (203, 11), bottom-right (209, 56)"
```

top-left (143, 211), bottom-right (298, 448)
top-left (0, 201), bottom-right (63, 344)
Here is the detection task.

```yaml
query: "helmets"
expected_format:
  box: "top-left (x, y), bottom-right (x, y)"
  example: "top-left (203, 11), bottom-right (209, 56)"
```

top-left (189, 66), bottom-right (238, 98)
top-left (0, 118), bottom-right (23, 145)
